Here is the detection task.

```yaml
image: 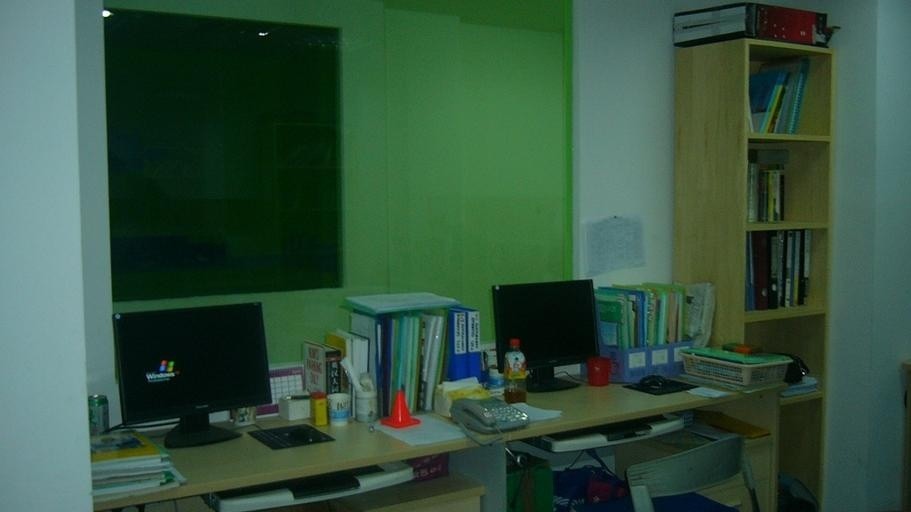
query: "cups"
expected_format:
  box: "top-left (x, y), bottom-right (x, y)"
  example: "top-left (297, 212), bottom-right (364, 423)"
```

top-left (587, 356), bottom-right (619, 386)
top-left (326, 393), bottom-right (351, 427)
top-left (233, 406), bottom-right (257, 425)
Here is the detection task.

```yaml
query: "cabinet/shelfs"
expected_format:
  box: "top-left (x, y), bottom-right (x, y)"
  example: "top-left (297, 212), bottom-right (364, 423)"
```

top-left (671, 38), bottom-right (834, 512)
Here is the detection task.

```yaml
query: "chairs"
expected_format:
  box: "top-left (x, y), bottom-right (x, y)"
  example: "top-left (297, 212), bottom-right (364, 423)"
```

top-left (626, 434), bottom-right (759, 510)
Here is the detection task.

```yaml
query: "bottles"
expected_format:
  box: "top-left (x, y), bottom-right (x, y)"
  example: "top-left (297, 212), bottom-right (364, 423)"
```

top-left (504, 338), bottom-right (527, 404)
top-left (311, 391), bottom-right (328, 426)
top-left (88, 393), bottom-right (111, 437)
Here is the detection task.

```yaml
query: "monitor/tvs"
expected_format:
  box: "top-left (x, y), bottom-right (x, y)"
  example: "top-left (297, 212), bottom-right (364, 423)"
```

top-left (492, 279), bottom-right (597, 393)
top-left (112, 302), bottom-right (276, 448)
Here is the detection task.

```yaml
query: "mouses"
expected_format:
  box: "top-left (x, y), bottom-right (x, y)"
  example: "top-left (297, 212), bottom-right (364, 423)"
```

top-left (289, 428), bottom-right (318, 443)
top-left (640, 374), bottom-right (666, 388)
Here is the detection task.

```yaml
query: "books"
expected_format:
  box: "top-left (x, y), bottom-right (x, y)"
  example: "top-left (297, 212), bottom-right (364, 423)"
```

top-left (746, 230), bottom-right (816, 312)
top-left (746, 156), bottom-right (789, 228)
top-left (299, 288), bottom-right (460, 415)
top-left (747, 57), bottom-right (807, 137)
top-left (90, 430), bottom-right (187, 500)
top-left (592, 280), bottom-right (718, 347)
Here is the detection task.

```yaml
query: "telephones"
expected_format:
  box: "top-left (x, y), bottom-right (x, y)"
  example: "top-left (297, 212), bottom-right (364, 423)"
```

top-left (449, 397), bottom-right (530, 447)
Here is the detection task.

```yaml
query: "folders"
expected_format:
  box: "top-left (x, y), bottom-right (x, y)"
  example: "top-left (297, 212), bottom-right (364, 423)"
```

top-left (452, 305), bottom-right (482, 380)
top-left (446, 307), bottom-right (468, 380)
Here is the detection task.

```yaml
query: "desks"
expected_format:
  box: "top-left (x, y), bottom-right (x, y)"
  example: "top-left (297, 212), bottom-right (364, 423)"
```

top-left (93, 375), bottom-right (787, 511)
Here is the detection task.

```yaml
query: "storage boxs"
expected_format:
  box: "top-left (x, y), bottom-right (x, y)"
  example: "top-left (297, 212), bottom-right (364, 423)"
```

top-left (673, 4), bottom-right (828, 48)
